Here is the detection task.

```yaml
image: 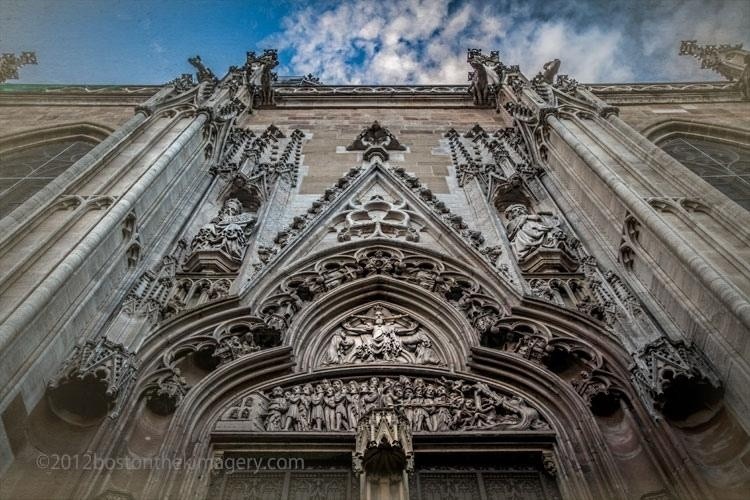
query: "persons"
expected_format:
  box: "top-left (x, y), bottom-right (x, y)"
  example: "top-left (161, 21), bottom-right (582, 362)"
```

top-left (209, 196), bottom-right (244, 232)
top-left (318, 306), bottom-right (441, 365)
top-left (262, 378), bottom-right (452, 432)
top-left (503, 202), bottom-right (542, 242)
